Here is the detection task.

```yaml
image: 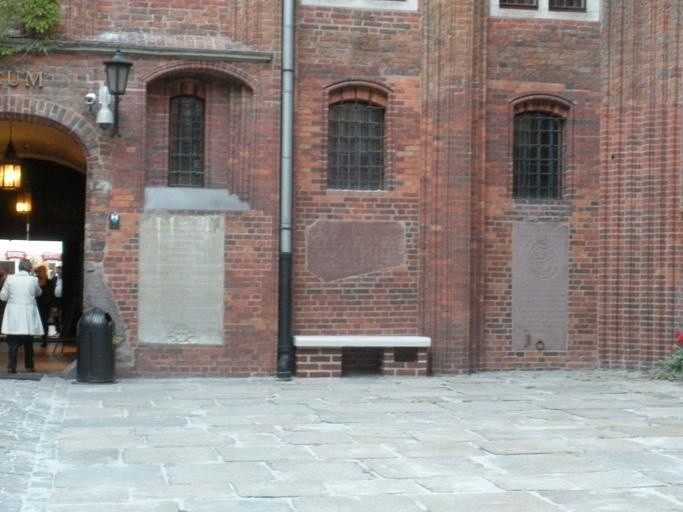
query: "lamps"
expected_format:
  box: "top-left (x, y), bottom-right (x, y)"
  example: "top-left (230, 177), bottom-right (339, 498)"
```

top-left (97, 48), bottom-right (133, 139)
top-left (0, 121), bottom-right (33, 214)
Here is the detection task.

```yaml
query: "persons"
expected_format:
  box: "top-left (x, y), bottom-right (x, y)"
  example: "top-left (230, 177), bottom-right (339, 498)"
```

top-left (35, 266), bottom-right (53, 347)
top-left (0, 258), bottom-right (45, 372)
top-left (49, 267), bottom-right (62, 335)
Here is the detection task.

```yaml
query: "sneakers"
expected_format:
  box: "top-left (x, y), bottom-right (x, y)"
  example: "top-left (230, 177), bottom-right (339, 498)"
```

top-left (8, 366), bottom-right (33, 373)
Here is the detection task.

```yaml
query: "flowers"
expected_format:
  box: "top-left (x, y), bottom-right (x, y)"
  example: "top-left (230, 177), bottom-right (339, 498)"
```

top-left (662, 330), bottom-right (683, 371)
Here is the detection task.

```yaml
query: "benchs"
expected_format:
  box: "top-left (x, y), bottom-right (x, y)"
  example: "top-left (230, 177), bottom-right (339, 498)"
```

top-left (292, 335), bottom-right (432, 377)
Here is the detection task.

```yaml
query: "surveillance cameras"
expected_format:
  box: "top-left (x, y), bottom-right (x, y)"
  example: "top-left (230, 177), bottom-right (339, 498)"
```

top-left (83, 93), bottom-right (96, 110)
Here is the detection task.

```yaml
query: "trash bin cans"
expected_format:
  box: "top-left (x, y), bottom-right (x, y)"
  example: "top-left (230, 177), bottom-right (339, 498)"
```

top-left (76, 308), bottom-right (115, 384)
